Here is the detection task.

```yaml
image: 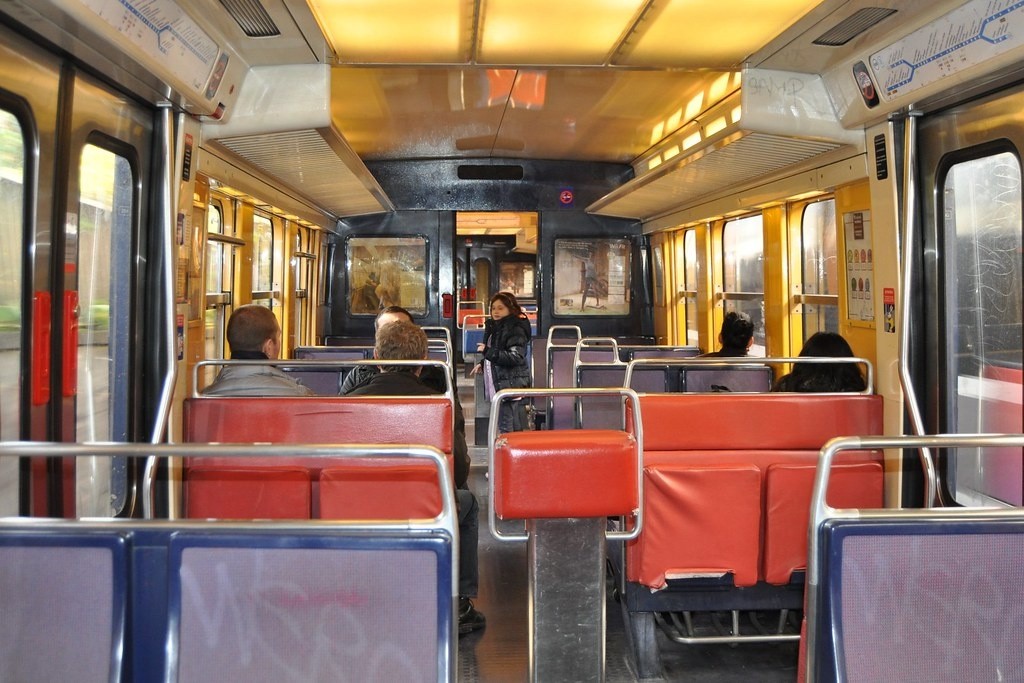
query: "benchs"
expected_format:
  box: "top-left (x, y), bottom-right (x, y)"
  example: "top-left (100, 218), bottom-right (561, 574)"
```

top-left (0, 300), bottom-right (1024, 683)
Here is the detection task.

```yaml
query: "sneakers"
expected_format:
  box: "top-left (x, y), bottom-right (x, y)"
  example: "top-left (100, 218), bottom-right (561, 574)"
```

top-left (459, 599), bottom-right (486, 633)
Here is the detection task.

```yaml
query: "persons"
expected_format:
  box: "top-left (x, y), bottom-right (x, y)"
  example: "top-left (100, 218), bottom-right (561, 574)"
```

top-left (470, 292), bottom-right (532, 480)
top-left (200, 304), bottom-right (319, 398)
top-left (338, 305), bottom-right (467, 438)
top-left (770, 331), bottom-right (877, 395)
top-left (345, 321), bottom-right (486, 635)
top-left (363, 271), bottom-right (380, 310)
top-left (572, 250), bottom-right (600, 311)
top-left (696, 310), bottom-right (759, 358)
